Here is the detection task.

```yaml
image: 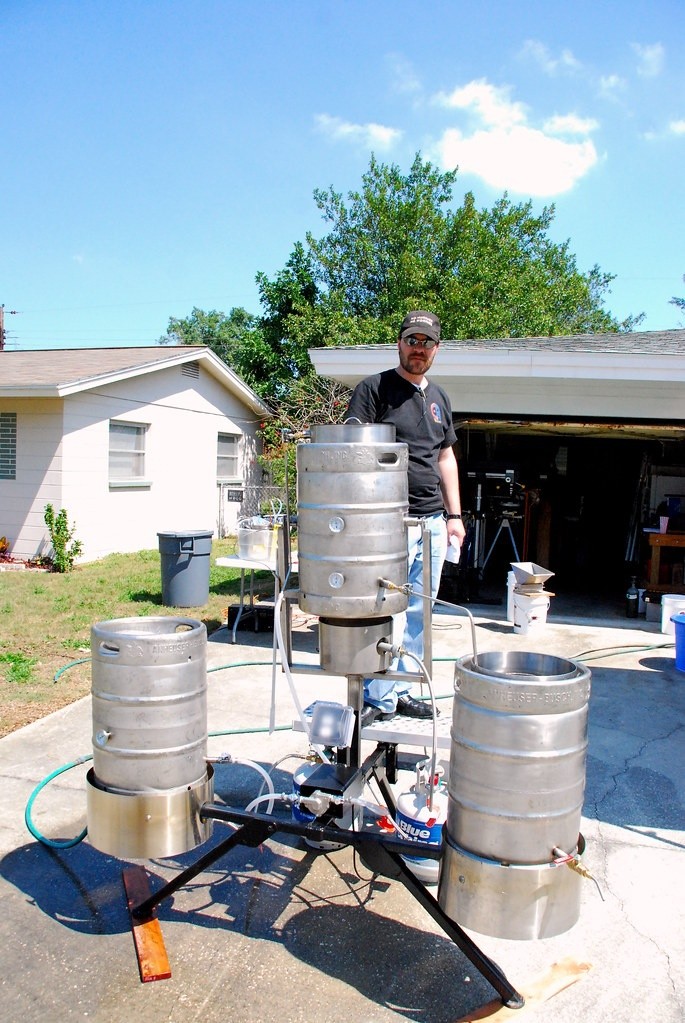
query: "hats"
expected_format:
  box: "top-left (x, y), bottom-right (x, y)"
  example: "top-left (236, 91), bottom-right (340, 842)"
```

top-left (398, 310), bottom-right (440, 342)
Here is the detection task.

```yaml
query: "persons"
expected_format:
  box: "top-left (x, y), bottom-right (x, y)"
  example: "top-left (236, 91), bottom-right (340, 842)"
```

top-left (345, 309), bottom-right (466, 728)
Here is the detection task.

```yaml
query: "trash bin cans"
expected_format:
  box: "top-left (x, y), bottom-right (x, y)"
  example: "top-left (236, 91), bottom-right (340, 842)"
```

top-left (156, 530), bottom-right (215, 608)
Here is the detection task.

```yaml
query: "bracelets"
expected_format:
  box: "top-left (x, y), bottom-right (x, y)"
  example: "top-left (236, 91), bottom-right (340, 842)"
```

top-left (446, 514), bottom-right (463, 520)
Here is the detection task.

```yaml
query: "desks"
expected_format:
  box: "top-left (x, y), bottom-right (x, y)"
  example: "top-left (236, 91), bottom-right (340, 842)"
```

top-left (643, 532), bottom-right (685, 599)
top-left (217, 551), bottom-right (299, 645)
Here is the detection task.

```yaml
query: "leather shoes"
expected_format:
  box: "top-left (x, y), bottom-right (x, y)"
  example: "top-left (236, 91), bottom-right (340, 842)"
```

top-left (361, 700), bottom-right (395, 726)
top-left (396, 695), bottom-right (440, 718)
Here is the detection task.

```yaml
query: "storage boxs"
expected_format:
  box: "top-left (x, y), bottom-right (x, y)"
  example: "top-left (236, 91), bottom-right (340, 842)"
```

top-left (227, 604), bottom-right (274, 634)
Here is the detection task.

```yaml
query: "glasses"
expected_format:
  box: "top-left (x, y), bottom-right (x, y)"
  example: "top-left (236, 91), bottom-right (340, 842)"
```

top-left (418, 387), bottom-right (427, 418)
top-left (400, 336), bottom-right (437, 349)
